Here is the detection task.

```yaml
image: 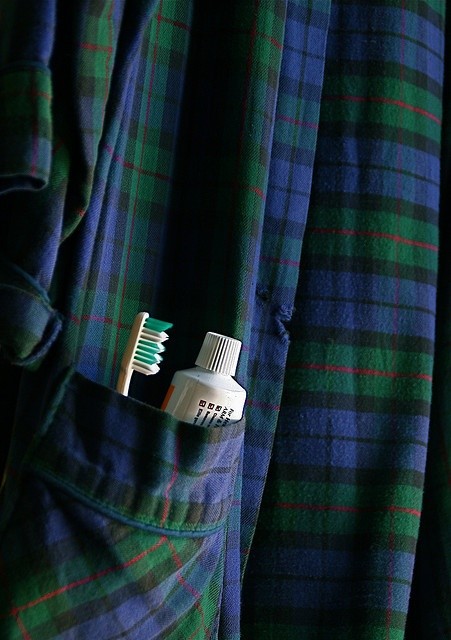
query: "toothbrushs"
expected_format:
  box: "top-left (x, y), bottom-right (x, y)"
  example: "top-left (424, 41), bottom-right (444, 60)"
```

top-left (116, 311), bottom-right (175, 397)
